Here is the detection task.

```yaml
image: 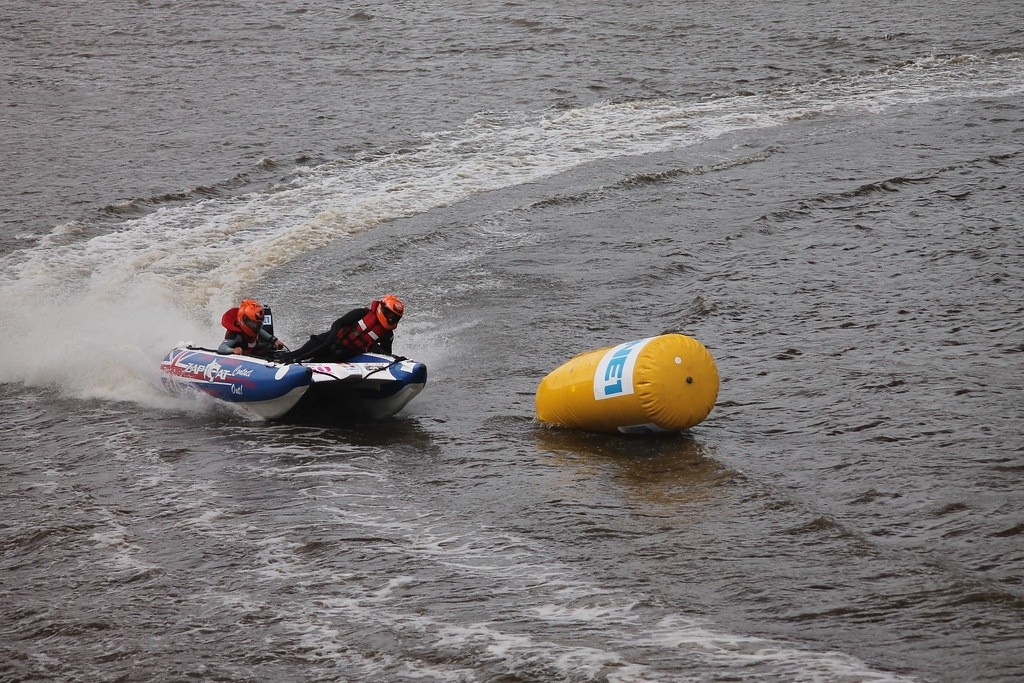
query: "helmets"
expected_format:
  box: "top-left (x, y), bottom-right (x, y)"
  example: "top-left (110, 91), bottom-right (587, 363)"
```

top-left (376, 295), bottom-right (404, 329)
top-left (236, 298), bottom-right (264, 337)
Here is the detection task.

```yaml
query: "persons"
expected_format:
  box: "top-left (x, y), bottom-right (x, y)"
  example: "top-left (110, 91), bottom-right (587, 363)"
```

top-left (281, 293), bottom-right (404, 363)
top-left (217, 298), bottom-right (288, 356)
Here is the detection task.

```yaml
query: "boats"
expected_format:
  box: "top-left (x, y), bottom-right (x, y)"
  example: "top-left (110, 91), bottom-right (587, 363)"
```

top-left (157, 343), bottom-right (428, 432)
